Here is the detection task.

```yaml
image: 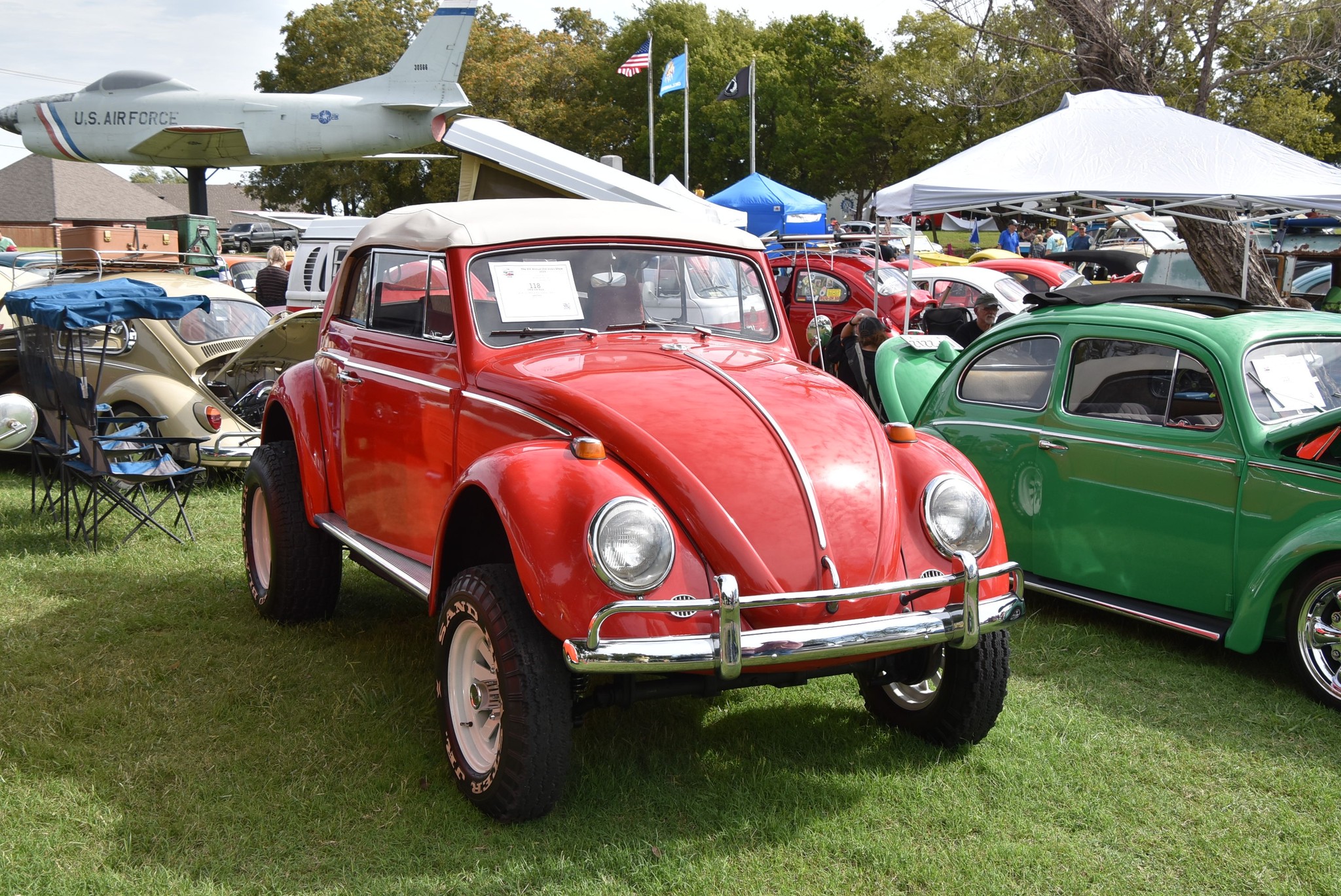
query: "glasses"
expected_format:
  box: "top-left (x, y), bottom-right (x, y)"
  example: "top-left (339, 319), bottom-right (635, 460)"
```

top-left (1025, 229), bottom-right (1028, 230)
top-left (976, 307), bottom-right (999, 312)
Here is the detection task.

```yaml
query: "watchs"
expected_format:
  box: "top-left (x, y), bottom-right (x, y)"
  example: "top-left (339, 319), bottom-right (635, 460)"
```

top-left (849, 316), bottom-right (857, 326)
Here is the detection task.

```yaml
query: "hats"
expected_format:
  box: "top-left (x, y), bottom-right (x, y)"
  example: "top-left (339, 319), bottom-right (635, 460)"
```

top-left (1107, 217), bottom-right (1118, 223)
top-left (974, 292), bottom-right (1003, 309)
top-left (1007, 219), bottom-right (1020, 226)
top-left (858, 316), bottom-right (887, 338)
top-left (831, 221), bottom-right (876, 233)
top-left (6, 245), bottom-right (18, 252)
top-left (1043, 228), bottom-right (1052, 237)
top-left (1078, 223), bottom-right (1086, 228)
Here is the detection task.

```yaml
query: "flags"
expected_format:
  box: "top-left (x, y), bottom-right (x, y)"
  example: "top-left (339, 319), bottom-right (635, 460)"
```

top-left (659, 53), bottom-right (686, 97)
top-left (617, 38), bottom-right (650, 77)
top-left (716, 65), bottom-right (751, 101)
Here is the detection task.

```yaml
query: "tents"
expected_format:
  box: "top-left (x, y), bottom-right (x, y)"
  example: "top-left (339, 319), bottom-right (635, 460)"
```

top-left (874, 89), bottom-right (1341, 335)
top-left (703, 171), bottom-right (827, 277)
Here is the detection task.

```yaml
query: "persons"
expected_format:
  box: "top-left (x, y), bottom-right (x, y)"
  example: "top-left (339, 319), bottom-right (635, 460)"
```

top-left (1029, 227), bottom-right (1038, 242)
top-left (827, 217), bottom-right (836, 234)
top-left (905, 244), bottom-right (910, 254)
top-left (1103, 217), bottom-right (1121, 244)
top-left (953, 292), bottom-right (999, 353)
top-left (1028, 234), bottom-right (1045, 258)
top-left (1020, 226), bottom-right (1033, 242)
top-left (1071, 223), bottom-right (1094, 271)
top-left (1024, 227), bottom-right (1031, 242)
top-left (1045, 228), bottom-right (1068, 264)
top-left (870, 240), bottom-right (897, 262)
top-left (256, 246), bottom-right (290, 307)
top-left (838, 308), bottom-right (877, 399)
top-left (843, 224), bottom-right (853, 233)
top-left (833, 220), bottom-right (846, 233)
top-left (997, 219), bottom-right (1021, 256)
top-left (840, 313), bottom-right (890, 425)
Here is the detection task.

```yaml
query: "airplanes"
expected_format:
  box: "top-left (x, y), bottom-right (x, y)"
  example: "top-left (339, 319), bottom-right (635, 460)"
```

top-left (1, 0), bottom-right (475, 169)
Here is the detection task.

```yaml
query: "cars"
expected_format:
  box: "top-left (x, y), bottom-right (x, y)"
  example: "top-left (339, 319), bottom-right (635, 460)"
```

top-left (0, 205), bottom-right (1341, 493)
top-left (874, 282), bottom-right (1341, 706)
top-left (241, 198), bottom-right (1051, 829)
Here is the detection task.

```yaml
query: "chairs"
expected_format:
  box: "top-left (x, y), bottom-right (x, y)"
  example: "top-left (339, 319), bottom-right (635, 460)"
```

top-left (589, 283), bottom-right (666, 334)
top-left (18, 353), bottom-right (169, 523)
top-left (417, 295), bottom-right (457, 348)
top-left (47, 368), bottom-right (212, 557)
